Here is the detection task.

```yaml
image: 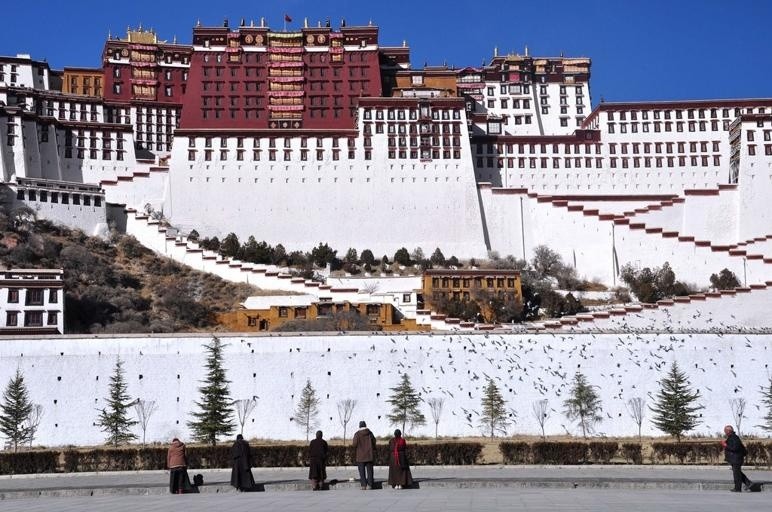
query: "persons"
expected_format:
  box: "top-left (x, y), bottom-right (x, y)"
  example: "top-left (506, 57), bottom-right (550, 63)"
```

top-left (307, 430), bottom-right (328, 490)
top-left (352, 421), bottom-right (377, 489)
top-left (228, 433), bottom-right (255, 492)
top-left (721, 425), bottom-right (761, 493)
top-left (386, 428), bottom-right (408, 489)
top-left (168, 438), bottom-right (188, 494)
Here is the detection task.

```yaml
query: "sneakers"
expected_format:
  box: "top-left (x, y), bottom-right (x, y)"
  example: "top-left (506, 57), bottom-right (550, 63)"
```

top-left (394, 484), bottom-right (403, 490)
top-left (360, 484), bottom-right (372, 490)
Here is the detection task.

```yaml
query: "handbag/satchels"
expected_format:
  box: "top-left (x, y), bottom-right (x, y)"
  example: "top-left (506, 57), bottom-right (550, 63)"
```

top-left (193, 474), bottom-right (203, 485)
